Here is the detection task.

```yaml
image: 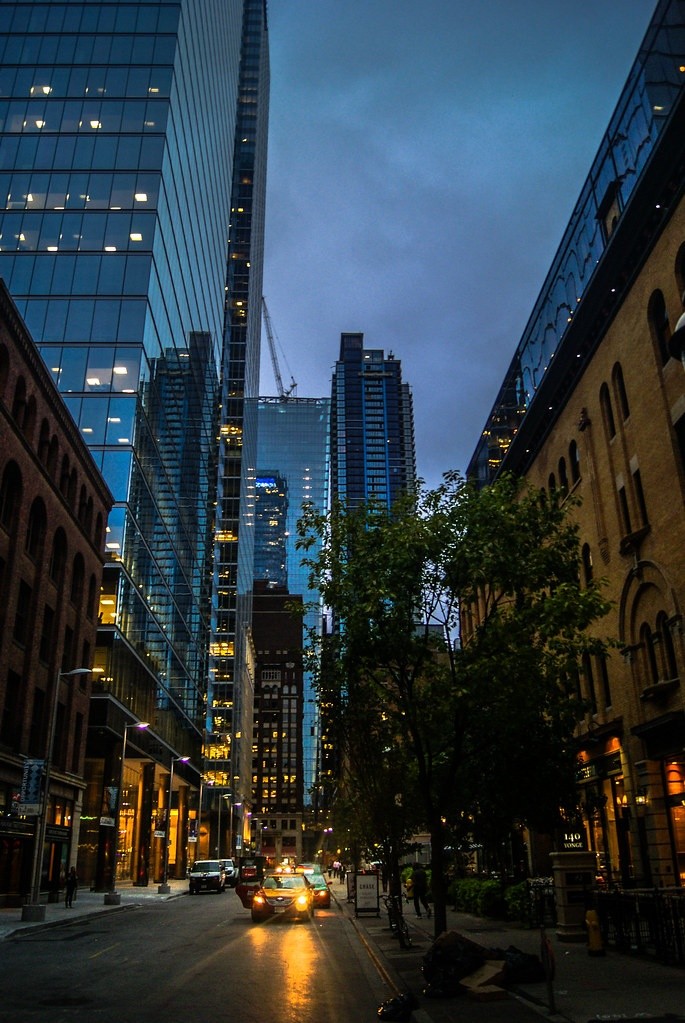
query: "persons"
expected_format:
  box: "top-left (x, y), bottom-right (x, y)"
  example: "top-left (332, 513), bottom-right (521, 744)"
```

top-left (381, 865), bottom-right (388, 892)
top-left (409, 863), bottom-right (431, 919)
top-left (328, 864), bottom-right (345, 884)
top-left (65, 867), bottom-right (77, 908)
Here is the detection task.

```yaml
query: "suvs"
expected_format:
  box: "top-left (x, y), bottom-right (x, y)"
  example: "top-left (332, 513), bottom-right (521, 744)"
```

top-left (219, 859), bottom-right (239, 888)
top-left (190, 860), bottom-right (226, 893)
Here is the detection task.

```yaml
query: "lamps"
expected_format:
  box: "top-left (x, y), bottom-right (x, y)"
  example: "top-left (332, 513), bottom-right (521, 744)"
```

top-left (578, 416), bottom-right (590, 432)
top-left (635, 784), bottom-right (649, 818)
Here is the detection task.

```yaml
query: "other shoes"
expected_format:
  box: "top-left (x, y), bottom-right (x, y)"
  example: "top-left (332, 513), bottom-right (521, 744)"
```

top-left (415, 915), bottom-right (422, 919)
top-left (427, 908), bottom-right (431, 919)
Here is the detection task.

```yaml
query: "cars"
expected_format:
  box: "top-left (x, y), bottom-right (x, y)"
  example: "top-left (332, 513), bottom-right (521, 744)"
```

top-left (303, 873), bottom-right (333, 908)
top-left (295, 865), bottom-right (315, 873)
top-left (275, 866), bottom-right (292, 872)
top-left (235, 873), bottom-right (316, 921)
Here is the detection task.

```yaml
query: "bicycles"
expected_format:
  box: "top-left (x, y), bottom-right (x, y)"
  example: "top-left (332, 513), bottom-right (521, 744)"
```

top-left (378, 893), bottom-right (413, 950)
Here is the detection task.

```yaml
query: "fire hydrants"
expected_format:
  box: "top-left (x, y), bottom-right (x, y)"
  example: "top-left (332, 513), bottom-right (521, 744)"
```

top-left (404, 878), bottom-right (414, 899)
top-left (584, 909), bottom-right (603, 956)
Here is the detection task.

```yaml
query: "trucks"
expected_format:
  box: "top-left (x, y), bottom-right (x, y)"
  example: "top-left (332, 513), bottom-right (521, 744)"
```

top-left (239, 857), bottom-right (266, 882)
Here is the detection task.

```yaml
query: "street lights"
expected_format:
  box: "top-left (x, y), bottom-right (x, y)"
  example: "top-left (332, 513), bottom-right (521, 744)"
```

top-left (196, 780), bottom-right (214, 858)
top-left (163, 756), bottom-right (190, 885)
top-left (217, 793), bottom-right (231, 856)
top-left (249, 818), bottom-right (259, 844)
top-left (242, 812), bottom-right (252, 840)
top-left (230, 803), bottom-right (241, 857)
top-left (111, 722), bottom-right (150, 888)
top-left (261, 826), bottom-right (267, 848)
top-left (256, 823), bottom-right (263, 851)
top-left (30, 668), bottom-right (92, 903)
